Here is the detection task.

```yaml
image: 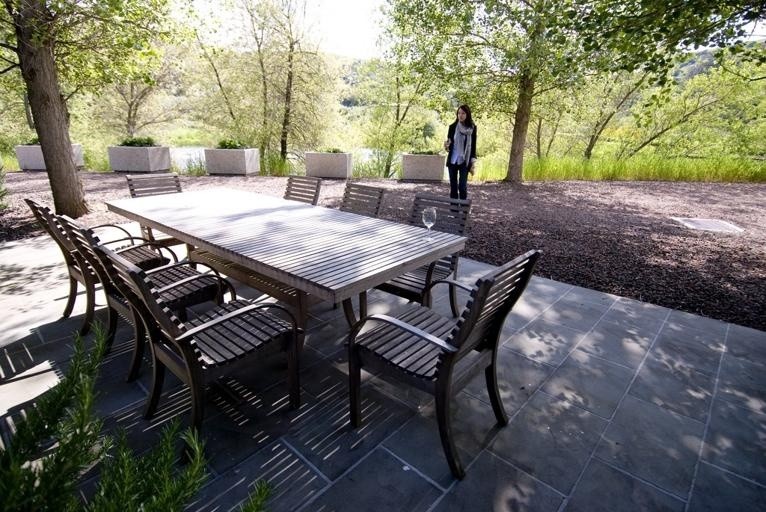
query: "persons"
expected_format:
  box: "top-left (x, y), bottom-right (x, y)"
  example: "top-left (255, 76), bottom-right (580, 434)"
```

top-left (445, 103), bottom-right (479, 219)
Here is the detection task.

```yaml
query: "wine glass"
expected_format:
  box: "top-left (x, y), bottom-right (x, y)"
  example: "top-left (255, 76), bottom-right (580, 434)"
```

top-left (422, 208), bottom-right (437, 241)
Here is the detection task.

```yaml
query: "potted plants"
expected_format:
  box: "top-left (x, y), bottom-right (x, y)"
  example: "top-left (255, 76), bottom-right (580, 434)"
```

top-left (204, 139), bottom-right (261, 177)
top-left (107, 136), bottom-right (171, 173)
top-left (13, 137), bottom-right (83, 171)
top-left (399, 142), bottom-right (445, 180)
top-left (305, 146), bottom-right (353, 179)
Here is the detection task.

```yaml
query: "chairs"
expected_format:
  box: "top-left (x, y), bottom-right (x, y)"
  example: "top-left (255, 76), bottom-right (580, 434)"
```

top-left (283, 174), bottom-right (322, 206)
top-left (357, 192), bottom-right (472, 319)
top-left (347, 249), bottom-right (544, 481)
top-left (23, 197), bottom-right (307, 461)
top-left (339, 182), bottom-right (384, 218)
top-left (125, 171), bottom-right (198, 270)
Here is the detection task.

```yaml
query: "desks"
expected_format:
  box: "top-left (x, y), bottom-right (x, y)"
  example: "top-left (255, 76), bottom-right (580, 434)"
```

top-left (105, 186), bottom-right (468, 395)
